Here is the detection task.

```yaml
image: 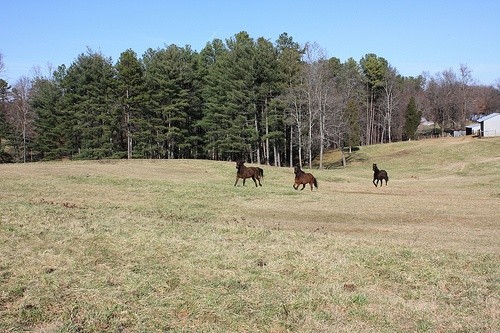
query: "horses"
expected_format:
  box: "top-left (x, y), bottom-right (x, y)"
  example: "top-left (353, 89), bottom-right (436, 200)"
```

top-left (372, 164), bottom-right (390, 186)
top-left (233, 159), bottom-right (264, 187)
top-left (293, 164), bottom-right (318, 191)
